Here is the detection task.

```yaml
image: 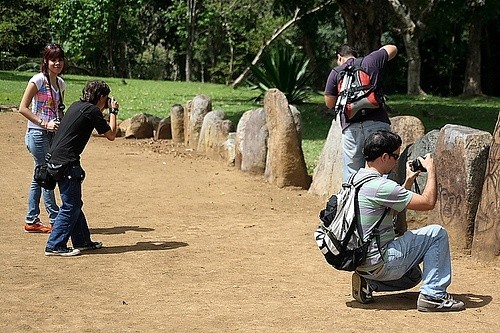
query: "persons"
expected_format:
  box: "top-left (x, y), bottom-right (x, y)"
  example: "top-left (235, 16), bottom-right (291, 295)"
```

top-left (346, 131), bottom-right (466, 312)
top-left (43, 80), bottom-right (119, 256)
top-left (19, 43), bottom-right (67, 233)
top-left (325, 45), bottom-right (399, 236)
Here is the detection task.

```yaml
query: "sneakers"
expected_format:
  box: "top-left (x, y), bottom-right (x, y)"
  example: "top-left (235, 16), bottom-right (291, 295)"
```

top-left (25, 218), bottom-right (53, 233)
top-left (75, 242), bottom-right (102, 250)
top-left (417, 293), bottom-right (465, 312)
top-left (352, 273), bottom-right (375, 304)
top-left (45, 247), bottom-right (80, 256)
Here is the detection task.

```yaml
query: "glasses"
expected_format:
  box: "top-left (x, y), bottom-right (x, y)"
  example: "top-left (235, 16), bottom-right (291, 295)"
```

top-left (388, 152), bottom-right (399, 160)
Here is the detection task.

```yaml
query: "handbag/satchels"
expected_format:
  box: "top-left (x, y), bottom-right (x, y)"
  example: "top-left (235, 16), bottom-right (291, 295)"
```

top-left (60, 160), bottom-right (85, 182)
top-left (35, 165), bottom-right (56, 191)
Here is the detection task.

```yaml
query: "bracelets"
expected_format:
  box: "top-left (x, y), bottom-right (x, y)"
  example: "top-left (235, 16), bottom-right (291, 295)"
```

top-left (45, 121), bottom-right (48, 129)
top-left (108, 107), bottom-right (118, 115)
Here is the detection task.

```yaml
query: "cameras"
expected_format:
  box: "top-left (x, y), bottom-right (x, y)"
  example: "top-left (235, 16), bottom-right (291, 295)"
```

top-left (56, 116), bottom-right (64, 127)
top-left (410, 156), bottom-right (427, 172)
top-left (105, 97), bottom-right (116, 108)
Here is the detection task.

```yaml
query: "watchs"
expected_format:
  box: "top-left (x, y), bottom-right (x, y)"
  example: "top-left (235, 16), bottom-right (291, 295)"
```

top-left (37, 118), bottom-right (43, 126)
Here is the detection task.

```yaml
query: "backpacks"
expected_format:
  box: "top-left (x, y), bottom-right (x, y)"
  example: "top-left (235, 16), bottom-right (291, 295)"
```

top-left (331, 57), bottom-right (383, 123)
top-left (313, 171), bottom-right (389, 271)
